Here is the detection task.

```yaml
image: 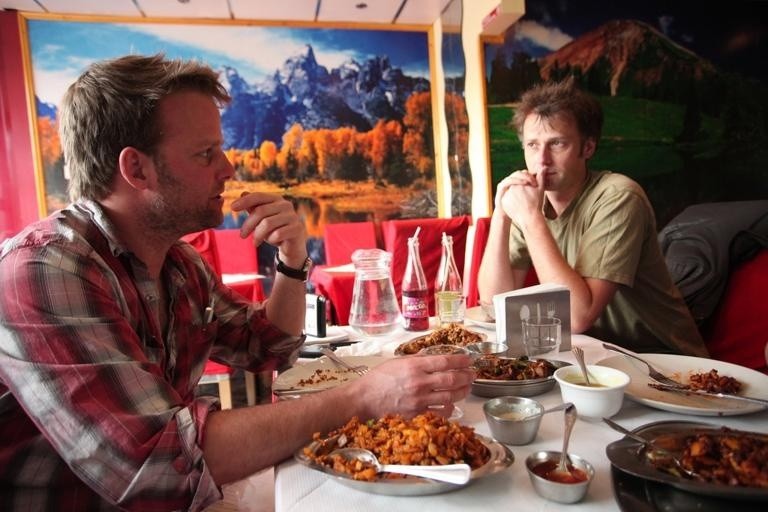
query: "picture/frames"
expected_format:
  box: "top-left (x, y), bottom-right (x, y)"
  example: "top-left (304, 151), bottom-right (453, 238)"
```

top-left (479, 0), bottom-right (768, 233)
top-left (16, 11), bottom-right (442, 298)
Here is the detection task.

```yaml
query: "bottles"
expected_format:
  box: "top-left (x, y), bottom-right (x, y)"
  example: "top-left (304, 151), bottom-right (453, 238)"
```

top-left (348, 249), bottom-right (402, 336)
top-left (435, 236), bottom-right (463, 323)
top-left (402, 238), bottom-right (429, 330)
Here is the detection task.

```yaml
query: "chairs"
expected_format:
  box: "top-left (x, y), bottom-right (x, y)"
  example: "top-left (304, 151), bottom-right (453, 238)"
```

top-left (664, 253), bottom-right (767, 372)
top-left (323, 221), bottom-right (378, 270)
top-left (467, 217), bottom-right (539, 308)
top-left (214, 228), bottom-right (259, 275)
top-left (391, 213), bottom-right (470, 313)
top-left (177, 229), bottom-right (257, 411)
top-left (382, 220), bottom-right (397, 254)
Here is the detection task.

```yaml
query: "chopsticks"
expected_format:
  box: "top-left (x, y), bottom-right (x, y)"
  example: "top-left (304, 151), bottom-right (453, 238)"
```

top-left (294, 426), bottom-right (515, 497)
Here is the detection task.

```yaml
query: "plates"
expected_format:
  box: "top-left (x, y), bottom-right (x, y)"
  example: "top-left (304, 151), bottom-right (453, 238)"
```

top-left (466, 357), bottom-right (573, 398)
top-left (273, 346), bottom-right (458, 421)
top-left (611, 420), bottom-right (767, 512)
top-left (597, 353), bottom-right (768, 417)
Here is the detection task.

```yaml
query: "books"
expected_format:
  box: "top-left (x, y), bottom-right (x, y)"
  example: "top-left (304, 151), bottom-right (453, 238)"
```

top-left (493, 283), bottom-right (570, 358)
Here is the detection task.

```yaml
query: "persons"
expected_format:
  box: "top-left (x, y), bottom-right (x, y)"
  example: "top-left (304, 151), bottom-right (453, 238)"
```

top-left (0, 55), bottom-right (478, 512)
top-left (479, 81), bottom-right (710, 359)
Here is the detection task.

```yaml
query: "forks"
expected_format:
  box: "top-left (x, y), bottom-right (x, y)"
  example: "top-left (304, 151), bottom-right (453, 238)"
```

top-left (547, 302), bottom-right (556, 347)
top-left (320, 346), bottom-right (368, 377)
top-left (604, 344), bottom-right (684, 389)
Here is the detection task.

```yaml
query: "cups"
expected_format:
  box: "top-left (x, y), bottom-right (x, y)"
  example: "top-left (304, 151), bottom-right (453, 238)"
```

top-left (522, 317), bottom-right (562, 362)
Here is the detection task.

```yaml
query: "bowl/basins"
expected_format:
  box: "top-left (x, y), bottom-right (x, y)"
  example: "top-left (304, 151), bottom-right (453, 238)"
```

top-left (525, 450), bottom-right (595, 504)
top-left (554, 365), bottom-right (630, 424)
top-left (482, 395), bottom-right (545, 445)
top-left (466, 342), bottom-right (509, 356)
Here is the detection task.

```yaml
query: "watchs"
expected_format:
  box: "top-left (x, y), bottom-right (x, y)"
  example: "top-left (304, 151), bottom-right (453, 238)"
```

top-left (274, 252), bottom-right (312, 282)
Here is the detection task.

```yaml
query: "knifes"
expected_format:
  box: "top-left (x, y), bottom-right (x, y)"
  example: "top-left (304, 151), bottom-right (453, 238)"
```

top-left (537, 302), bottom-right (542, 348)
top-left (648, 384), bottom-right (767, 404)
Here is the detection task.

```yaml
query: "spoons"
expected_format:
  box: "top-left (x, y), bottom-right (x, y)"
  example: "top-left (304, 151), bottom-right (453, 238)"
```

top-left (330, 447), bottom-right (470, 485)
top-left (519, 304), bottom-right (530, 351)
